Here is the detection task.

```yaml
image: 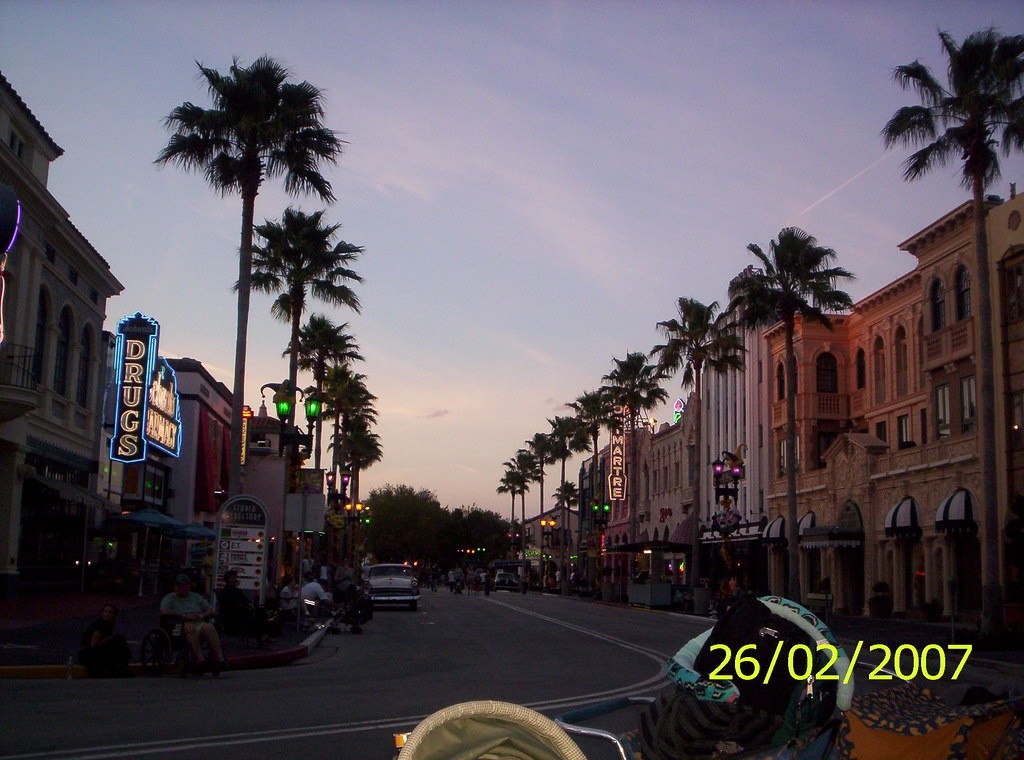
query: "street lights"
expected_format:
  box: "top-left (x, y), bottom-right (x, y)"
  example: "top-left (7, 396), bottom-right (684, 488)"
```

top-left (540, 515), bottom-right (558, 594)
top-left (711, 443), bottom-right (749, 617)
top-left (327, 469), bottom-right (371, 565)
top-left (592, 499), bottom-right (611, 600)
top-left (260, 379), bottom-right (324, 494)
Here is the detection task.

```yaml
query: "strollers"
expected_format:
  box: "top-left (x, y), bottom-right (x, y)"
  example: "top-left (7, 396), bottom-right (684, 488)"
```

top-left (327, 584), bottom-right (374, 635)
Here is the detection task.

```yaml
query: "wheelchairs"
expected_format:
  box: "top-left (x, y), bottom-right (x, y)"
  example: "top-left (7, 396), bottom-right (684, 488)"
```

top-left (140, 598), bottom-right (220, 678)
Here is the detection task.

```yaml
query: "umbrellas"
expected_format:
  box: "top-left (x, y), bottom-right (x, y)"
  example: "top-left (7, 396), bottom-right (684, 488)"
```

top-left (116, 507), bottom-right (186, 560)
top-left (163, 521), bottom-right (217, 556)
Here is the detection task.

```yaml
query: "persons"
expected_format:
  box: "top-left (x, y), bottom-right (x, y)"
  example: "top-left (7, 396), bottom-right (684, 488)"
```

top-left (79, 603), bottom-right (132, 679)
top-left (160, 574), bottom-right (226, 673)
top-left (280, 577), bottom-right (310, 616)
top-left (219, 569), bottom-right (287, 649)
top-left (301, 559), bottom-right (565, 635)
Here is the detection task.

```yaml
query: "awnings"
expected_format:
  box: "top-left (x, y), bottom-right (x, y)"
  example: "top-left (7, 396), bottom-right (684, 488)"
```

top-left (802, 526), bottom-right (865, 549)
top-left (795, 512), bottom-right (816, 544)
top-left (612, 540), bottom-right (693, 553)
top-left (32, 475), bottom-right (123, 513)
top-left (935, 488), bottom-right (979, 533)
top-left (763, 515), bottom-right (788, 547)
top-left (884, 498), bottom-right (923, 539)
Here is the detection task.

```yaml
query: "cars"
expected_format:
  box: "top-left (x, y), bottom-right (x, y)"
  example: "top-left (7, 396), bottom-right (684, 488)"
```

top-left (492, 572), bottom-right (518, 592)
top-left (364, 563), bottom-right (422, 611)
